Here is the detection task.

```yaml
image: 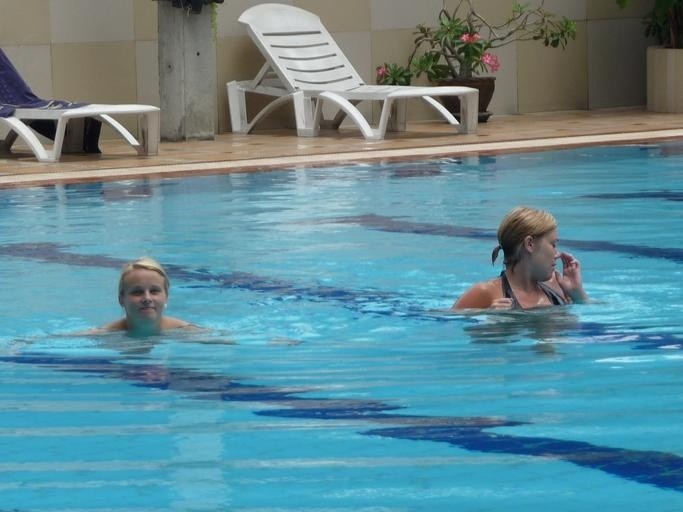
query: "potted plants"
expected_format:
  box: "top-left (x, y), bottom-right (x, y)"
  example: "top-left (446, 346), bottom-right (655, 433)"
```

top-left (616, 0), bottom-right (683, 114)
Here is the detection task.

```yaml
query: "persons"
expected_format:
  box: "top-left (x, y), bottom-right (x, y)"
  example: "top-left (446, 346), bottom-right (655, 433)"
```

top-left (101, 256), bottom-right (190, 332)
top-left (451, 205), bottom-right (590, 310)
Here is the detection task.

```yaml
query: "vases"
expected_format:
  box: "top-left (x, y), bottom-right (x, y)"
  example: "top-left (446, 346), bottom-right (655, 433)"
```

top-left (437, 76), bottom-right (496, 125)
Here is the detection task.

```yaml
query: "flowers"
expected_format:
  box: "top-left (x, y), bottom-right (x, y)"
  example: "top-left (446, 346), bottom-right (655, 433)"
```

top-left (376, 0), bottom-right (577, 86)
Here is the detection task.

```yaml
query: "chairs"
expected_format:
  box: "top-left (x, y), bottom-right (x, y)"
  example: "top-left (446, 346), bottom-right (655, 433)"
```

top-left (225, 2), bottom-right (480, 141)
top-left (0, 46), bottom-right (162, 163)
